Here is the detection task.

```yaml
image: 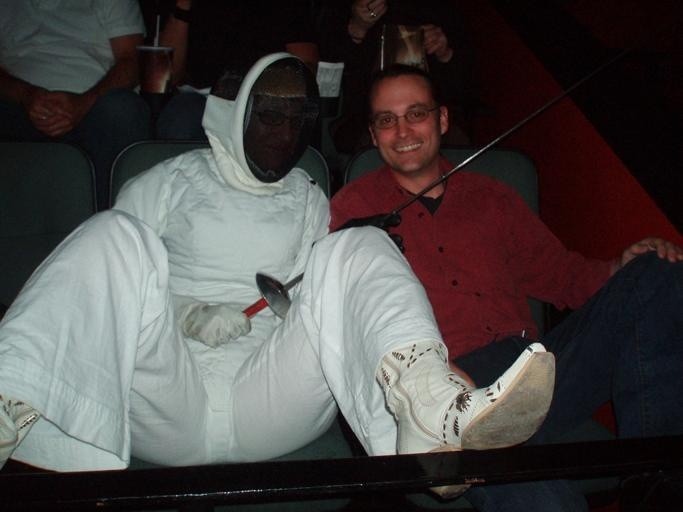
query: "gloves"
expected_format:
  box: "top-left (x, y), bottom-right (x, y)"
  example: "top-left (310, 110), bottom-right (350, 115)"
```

top-left (183, 305), bottom-right (250, 346)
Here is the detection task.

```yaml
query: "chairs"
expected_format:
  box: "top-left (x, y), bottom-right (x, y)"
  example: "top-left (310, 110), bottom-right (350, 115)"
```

top-left (106, 136), bottom-right (361, 512)
top-left (341, 143), bottom-right (626, 511)
top-left (0, 140), bottom-right (99, 318)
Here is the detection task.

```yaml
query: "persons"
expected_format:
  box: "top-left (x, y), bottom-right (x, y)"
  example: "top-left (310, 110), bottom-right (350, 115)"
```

top-left (0, 53), bottom-right (556, 501)
top-left (1, 2), bottom-right (151, 210)
top-left (332, 62), bottom-right (680, 511)
top-left (141, 0), bottom-right (324, 142)
top-left (316, 0), bottom-right (469, 153)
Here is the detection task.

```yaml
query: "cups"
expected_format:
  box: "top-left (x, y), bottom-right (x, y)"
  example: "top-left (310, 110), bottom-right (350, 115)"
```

top-left (394, 25), bottom-right (426, 68)
top-left (135, 47), bottom-right (175, 93)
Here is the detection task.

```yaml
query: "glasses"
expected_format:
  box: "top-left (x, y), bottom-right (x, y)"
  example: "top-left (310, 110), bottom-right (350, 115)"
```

top-left (374, 105), bottom-right (439, 129)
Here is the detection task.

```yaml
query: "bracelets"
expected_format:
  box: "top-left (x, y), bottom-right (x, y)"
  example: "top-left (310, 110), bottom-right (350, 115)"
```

top-left (169, 4), bottom-right (200, 26)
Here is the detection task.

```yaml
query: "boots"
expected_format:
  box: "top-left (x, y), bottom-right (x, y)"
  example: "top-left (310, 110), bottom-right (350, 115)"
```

top-left (2, 395), bottom-right (41, 466)
top-left (374, 341), bottom-right (557, 495)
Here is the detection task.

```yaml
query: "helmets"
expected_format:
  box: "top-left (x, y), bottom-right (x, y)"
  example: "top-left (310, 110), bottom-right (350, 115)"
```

top-left (203, 47), bottom-right (320, 195)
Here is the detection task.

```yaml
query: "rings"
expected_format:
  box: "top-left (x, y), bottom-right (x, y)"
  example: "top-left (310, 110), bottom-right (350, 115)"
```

top-left (370, 11), bottom-right (376, 17)
top-left (367, 7), bottom-right (371, 11)
